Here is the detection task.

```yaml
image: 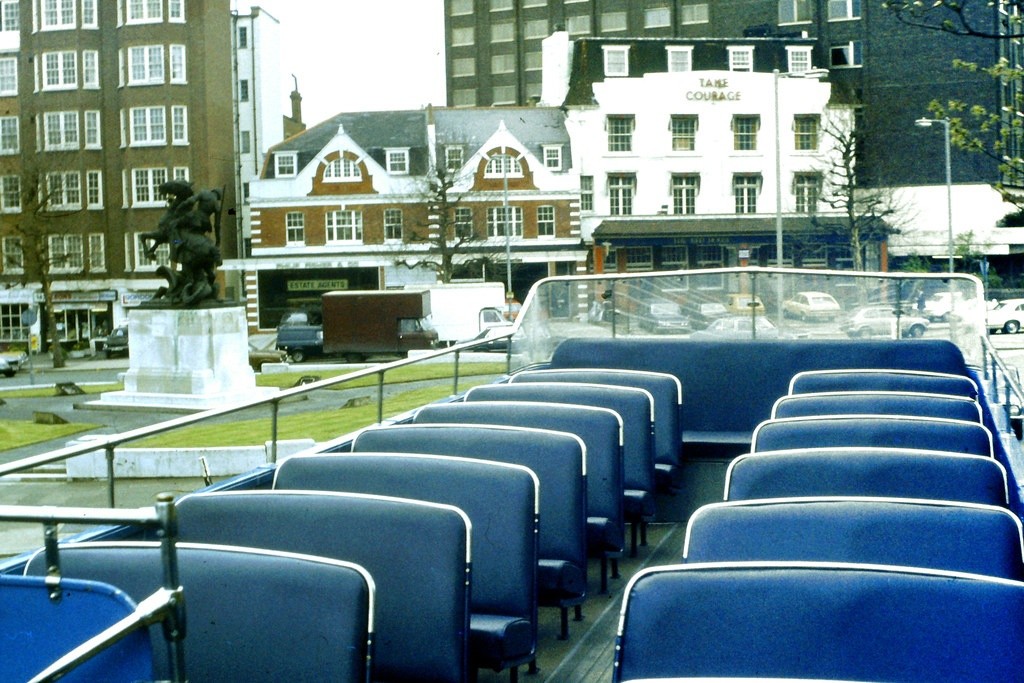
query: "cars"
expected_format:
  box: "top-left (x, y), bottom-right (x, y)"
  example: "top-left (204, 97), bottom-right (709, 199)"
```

top-left (279, 309), bottom-right (320, 328)
top-left (0, 350), bottom-right (29, 378)
top-left (639, 299), bottom-right (694, 336)
top-left (685, 302), bottom-right (734, 329)
top-left (720, 295), bottom-right (766, 316)
top-left (103, 326), bottom-right (129, 360)
top-left (782, 291), bottom-right (844, 324)
top-left (837, 289), bottom-right (979, 339)
top-left (505, 298), bottom-right (523, 325)
top-left (688, 317), bottom-right (780, 338)
top-left (786, 327), bottom-right (848, 340)
top-left (985, 298), bottom-right (1024, 335)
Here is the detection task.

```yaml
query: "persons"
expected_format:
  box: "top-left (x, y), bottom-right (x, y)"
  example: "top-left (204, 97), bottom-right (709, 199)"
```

top-left (915, 288), bottom-right (926, 311)
top-left (169, 188), bottom-right (223, 248)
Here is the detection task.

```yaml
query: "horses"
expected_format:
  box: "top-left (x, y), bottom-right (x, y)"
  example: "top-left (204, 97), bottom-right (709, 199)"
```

top-left (138, 177), bottom-right (223, 298)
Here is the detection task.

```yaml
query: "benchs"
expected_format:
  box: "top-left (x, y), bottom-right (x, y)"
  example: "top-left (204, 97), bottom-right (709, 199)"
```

top-left (0, 339), bottom-right (1024, 683)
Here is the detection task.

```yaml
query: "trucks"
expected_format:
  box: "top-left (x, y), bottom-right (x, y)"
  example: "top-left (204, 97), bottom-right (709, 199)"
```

top-left (405, 282), bottom-right (516, 345)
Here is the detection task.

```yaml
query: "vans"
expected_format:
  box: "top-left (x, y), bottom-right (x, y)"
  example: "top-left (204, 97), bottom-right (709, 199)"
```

top-left (323, 289), bottom-right (440, 365)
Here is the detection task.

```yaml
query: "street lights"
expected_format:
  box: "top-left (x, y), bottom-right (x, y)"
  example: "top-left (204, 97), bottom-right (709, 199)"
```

top-left (492, 154), bottom-right (515, 324)
top-left (913, 117), bottom-right (954, 284)
top-left (771, 68), bottom-right (786, 330)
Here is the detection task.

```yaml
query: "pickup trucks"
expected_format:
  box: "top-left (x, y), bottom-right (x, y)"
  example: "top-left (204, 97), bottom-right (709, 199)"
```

top-left (275, 326), bottom-right (323, 363)
